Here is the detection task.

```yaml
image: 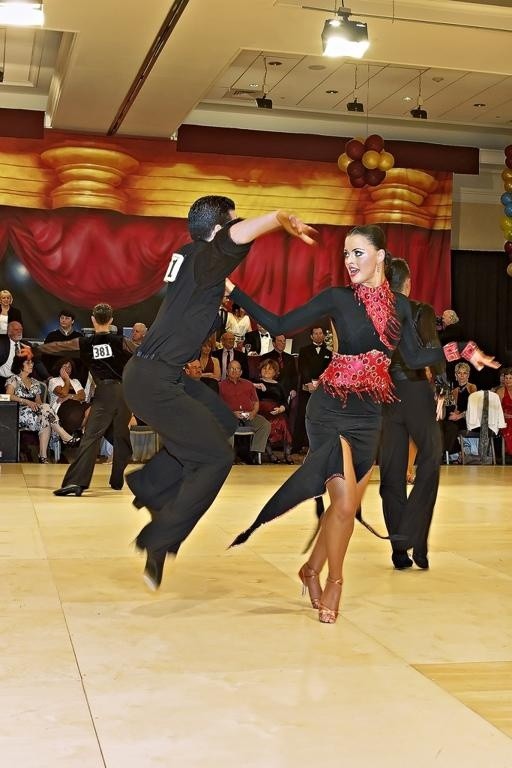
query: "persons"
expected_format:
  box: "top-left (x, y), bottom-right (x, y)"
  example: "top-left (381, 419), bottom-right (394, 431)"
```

top-left (225, 223), bottom-right (502, 624)
top-left (386, 258), bottom-right (446, 569)
top-left (122, 193), bottom-right (317, 589)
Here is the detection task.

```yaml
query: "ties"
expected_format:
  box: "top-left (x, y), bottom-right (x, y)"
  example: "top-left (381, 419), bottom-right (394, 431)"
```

top-left (226, 350), bottom-right (232, 381)
top-left (220, 307), bottom-right (225, 325)
top-left (278, 352), bottom-right (285, 370)
top-left (14, 343), bottom-right (20, 357)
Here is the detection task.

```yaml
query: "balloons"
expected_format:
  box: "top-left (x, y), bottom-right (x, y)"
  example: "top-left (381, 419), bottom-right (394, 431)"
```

top-left (339, 134), bottom-right (394, 189)
top-left (500, 145), bottom-right (512, 276)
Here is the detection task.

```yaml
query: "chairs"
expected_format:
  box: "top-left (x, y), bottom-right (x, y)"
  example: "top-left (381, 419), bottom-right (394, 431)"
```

top-left (1, 351), bottom-right (510, 466)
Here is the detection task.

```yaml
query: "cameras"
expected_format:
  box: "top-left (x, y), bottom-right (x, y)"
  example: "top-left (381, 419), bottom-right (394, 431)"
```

top-left (241, 412), bottom-right (249, 418)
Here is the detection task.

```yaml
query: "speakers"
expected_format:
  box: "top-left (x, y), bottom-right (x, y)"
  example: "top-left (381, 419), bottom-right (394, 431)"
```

top-left (0, 401), bottom-right (21, 463)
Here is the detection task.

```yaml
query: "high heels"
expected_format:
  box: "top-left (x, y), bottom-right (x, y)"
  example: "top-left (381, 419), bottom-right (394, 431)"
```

top-left (109, 479), bottom-right (125, 491)
top-left (318, 574), bottom-right (345, 624)
top-left (136, 525), bottom-right (169, 592)
top-left (62, 437), bottom-right (81, 448)
top-left (53, 482), bottom-right (84, 498)
top-left (37, 452), bottom-right (50, 464)
top-left (284, 450), bottom-right (296, 467)
top-left (298, 562), bottom-right (324, 610)
top-left (132, 494), bottom-right (162, 519)
top-left (267, 452), bottom-right (283, 465)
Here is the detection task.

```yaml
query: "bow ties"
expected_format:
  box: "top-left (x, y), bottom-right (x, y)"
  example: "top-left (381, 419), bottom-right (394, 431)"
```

top-left (313, 344), bottom-right (324, 349)
top-left (260, 333), bottom-right (269, 338)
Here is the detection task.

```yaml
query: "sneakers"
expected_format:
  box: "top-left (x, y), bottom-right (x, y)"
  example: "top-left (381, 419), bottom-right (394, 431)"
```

top-left (391, 552), bottom-right (414, 569)
top-left (412, 551), bottom-right (430, 570)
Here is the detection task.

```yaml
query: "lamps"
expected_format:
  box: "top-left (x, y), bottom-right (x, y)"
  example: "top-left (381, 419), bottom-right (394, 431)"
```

top-left (346, 64), bottom-right (366, 114)
top-left (255, 56), bottom-right (275, 112)
top-left (319, 0), bottom-right (371, 61)
top-left (410, 69), bottom-right (429, 119)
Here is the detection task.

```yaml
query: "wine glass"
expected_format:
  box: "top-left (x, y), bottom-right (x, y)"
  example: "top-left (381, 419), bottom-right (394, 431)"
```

top-left (244, 344), bottom-right (251, 355)
